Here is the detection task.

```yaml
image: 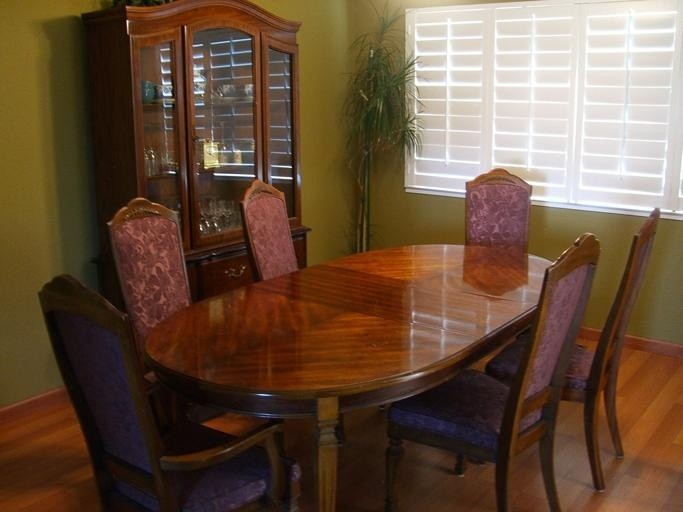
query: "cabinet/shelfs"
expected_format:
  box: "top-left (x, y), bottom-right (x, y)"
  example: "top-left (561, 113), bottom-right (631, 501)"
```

top-left (80, 0), bottom-right (311, 432)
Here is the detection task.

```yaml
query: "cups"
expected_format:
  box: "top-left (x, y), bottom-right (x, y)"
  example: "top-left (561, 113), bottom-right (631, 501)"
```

top-left (141, 80), bottom-right (156, 105)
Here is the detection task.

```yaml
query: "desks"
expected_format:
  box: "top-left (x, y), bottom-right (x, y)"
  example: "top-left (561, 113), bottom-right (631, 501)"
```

top-left (141, 235), bottom-right (553, 512)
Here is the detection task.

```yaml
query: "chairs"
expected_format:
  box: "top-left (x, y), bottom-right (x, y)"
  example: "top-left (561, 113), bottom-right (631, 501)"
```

top-left (39, 270), bottom-right (296, 512)
top-left (484, 209), bottom-right (660, 491)
top-left (464, 168), bottom-right (533, 254)
top-left (384, 234), bottom-right (601, 512)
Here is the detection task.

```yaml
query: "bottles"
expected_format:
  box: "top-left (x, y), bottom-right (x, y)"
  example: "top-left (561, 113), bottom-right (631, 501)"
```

top-left (197, 197), bottom-right (243, 236)
top-left (144, 144), bottom-right (158, 177)
